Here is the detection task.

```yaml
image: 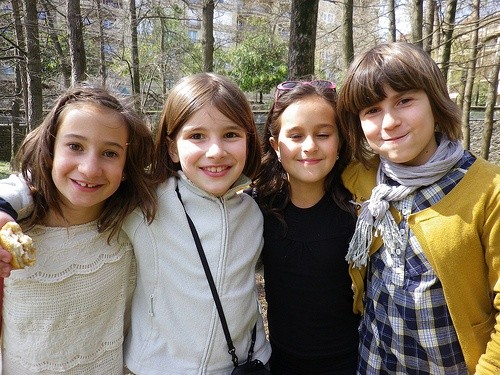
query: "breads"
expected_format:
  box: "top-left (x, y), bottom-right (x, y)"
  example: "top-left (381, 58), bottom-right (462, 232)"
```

top-left (0, 221), bottom-right (38, 269)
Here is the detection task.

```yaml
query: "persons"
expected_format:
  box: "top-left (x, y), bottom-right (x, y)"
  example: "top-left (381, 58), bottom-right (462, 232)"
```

top-left (0, 73), bottom-right (271, 375)
top-left (252, 41), bottom-right (500, 374)
top-left (0, 82), bottom-right (156, 375)
top-left (244, 79), bottom-right (361, 375)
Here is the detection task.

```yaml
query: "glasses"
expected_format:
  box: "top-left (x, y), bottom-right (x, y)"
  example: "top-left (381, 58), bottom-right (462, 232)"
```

top-left (276, 81), bottom-right (337, 105)
